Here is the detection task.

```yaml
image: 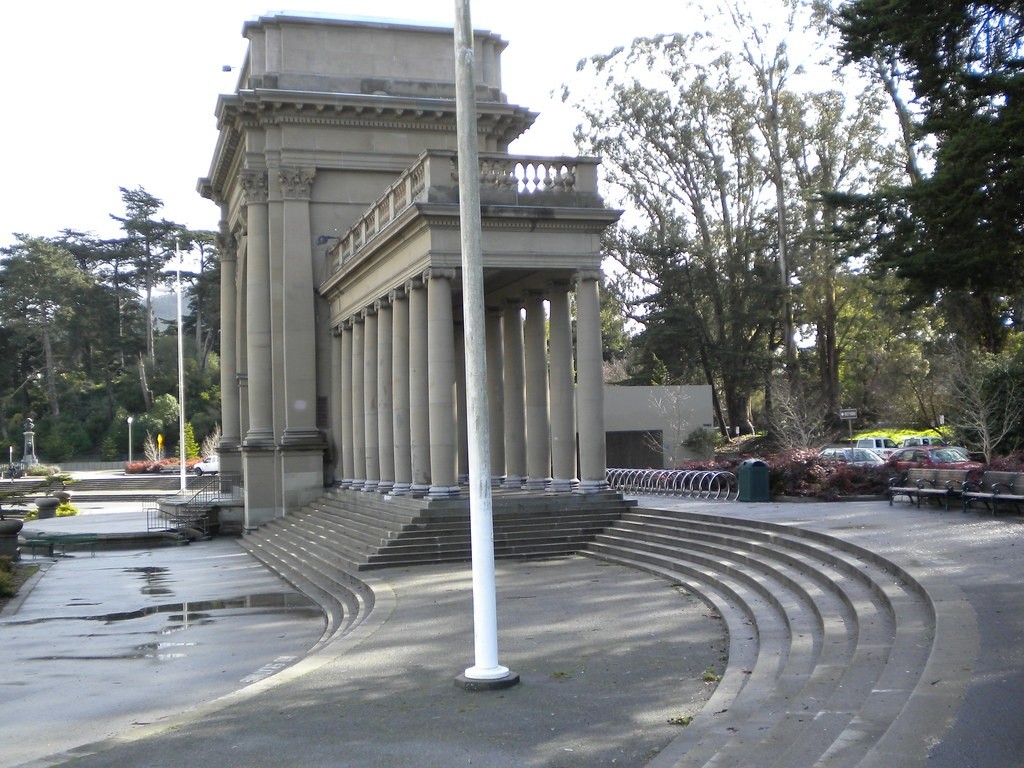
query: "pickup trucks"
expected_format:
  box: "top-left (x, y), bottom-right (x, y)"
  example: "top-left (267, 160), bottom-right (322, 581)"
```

top-left (885, 437), bottom-right (947, 458)
top-left (857, 438), bottom-right (897, 457)
top-left (193, 455), bottom-right (220, 476)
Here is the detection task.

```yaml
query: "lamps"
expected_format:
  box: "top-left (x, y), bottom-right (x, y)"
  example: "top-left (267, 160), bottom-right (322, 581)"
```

top-left (315, 235), bottom-right (342, 246)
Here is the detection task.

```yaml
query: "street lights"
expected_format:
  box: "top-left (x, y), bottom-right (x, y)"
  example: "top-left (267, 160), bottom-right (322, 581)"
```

top-left (127, 417), bottom-right (133, 464)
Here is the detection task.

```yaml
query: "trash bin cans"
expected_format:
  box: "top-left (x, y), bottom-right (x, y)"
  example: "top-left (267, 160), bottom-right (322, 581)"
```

top-left (736, 458), bottom-right (772, 502)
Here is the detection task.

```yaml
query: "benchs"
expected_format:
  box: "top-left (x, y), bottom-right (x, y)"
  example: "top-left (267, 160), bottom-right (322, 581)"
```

top-left (888, 468), bottom-right (972, 511)
top-left (962, 471), bottom-right (1024, 516)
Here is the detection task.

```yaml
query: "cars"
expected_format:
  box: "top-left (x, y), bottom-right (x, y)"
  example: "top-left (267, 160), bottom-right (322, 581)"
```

top-left (885, 446), bottom-right (985, 473)
top-left (819, 449), bottom-right (886, 471)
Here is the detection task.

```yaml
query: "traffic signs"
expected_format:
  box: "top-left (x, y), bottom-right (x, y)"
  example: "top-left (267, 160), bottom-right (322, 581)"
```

top-left (840, 409), bottom-right (856, 418)
